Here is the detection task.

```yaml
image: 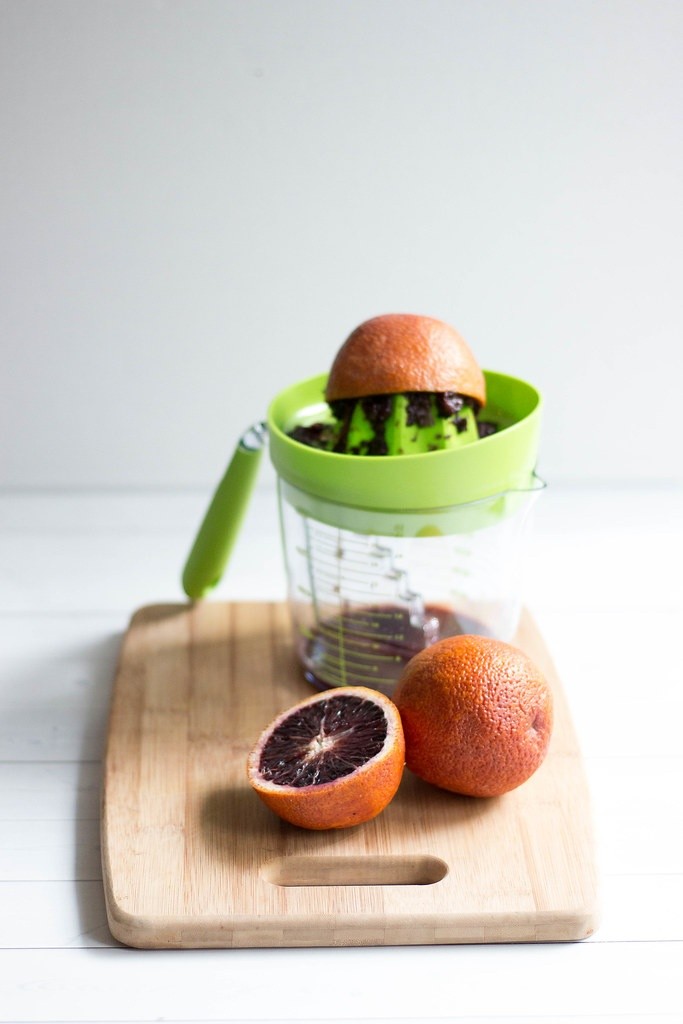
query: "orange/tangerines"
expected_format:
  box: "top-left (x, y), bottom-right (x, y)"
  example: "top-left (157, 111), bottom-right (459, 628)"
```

top-left (324, 313), bottom-right (487, 409)
top-left (394, 634), bottom-right (554, 796)
top-left (244, 685), bottom-right (406, 830)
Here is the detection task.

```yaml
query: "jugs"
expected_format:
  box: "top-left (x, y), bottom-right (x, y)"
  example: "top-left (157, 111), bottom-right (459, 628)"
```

top-left (184, 367), bottom-right (550, 701)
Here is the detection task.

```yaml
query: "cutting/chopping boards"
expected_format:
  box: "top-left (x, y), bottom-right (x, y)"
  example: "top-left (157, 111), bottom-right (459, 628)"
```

top-left (98, 601), bottom-right (593, 951)
